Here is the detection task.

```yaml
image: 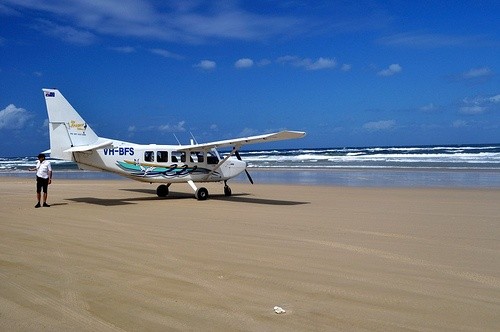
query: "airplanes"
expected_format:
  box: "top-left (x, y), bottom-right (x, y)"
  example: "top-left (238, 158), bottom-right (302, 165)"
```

top-left (39, 88), bottom-right (307, 201)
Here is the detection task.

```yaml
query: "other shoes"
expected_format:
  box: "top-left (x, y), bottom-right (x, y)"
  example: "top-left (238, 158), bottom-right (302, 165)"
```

top-left (43, 202), bottom-right (50, 207)
top-left (35, 202), bottom-right (40, 208)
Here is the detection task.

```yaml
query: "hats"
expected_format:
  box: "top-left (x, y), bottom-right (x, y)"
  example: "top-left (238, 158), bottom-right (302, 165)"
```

top-left (37, 154), bottom-right (45, 157)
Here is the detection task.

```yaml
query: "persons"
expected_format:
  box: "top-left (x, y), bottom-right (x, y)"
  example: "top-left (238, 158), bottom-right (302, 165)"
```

top-left (28, 154), bottom-right (53, 208)
